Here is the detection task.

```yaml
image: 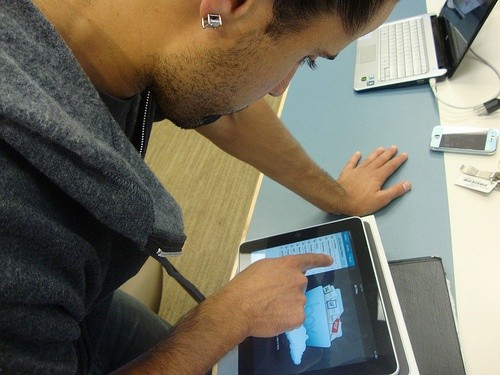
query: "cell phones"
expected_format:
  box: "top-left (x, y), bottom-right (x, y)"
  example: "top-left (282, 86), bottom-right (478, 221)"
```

top-left (429, 125), bottom-right (499, 155)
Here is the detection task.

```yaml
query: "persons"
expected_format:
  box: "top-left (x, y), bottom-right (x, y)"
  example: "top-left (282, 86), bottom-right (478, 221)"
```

top-left (0, 0), bottom-right (412, 375)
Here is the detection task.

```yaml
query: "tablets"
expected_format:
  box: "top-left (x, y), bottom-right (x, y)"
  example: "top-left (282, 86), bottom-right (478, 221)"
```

top-left (238, 213), bottom-right (400, 375)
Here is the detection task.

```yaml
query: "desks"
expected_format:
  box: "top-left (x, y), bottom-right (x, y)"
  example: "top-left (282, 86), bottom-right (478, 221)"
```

top-left (211, 1), bottom-right (499, 375)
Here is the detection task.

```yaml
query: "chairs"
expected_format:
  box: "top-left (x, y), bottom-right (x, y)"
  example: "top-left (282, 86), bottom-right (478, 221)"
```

top-left (118, 251), bottom-right (204, 317)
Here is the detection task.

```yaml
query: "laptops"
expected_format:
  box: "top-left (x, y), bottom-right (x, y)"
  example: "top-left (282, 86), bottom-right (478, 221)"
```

top-left (354, 0), bottom-right (497, 91)
